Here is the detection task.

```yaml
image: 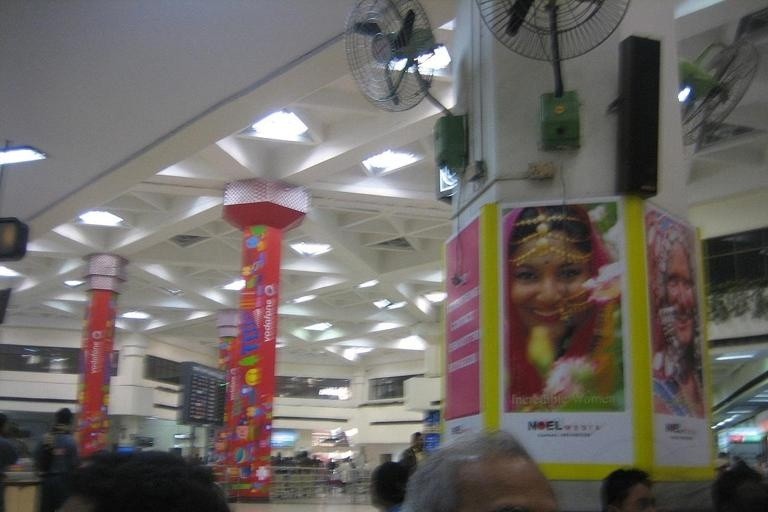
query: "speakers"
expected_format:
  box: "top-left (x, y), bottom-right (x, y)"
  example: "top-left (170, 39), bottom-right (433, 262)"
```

top-left (617, 34), bottom-right (660, 199)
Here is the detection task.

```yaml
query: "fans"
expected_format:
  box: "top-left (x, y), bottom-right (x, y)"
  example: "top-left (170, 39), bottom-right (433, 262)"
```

top-left (477, 0), bottom-right (632, 153)
top-left (345, 0), bottom-right (470, 172)
top-left (678, 41), bottom-right (759, 149)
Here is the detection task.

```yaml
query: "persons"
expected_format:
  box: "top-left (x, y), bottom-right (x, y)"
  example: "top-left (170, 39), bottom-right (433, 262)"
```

top-left (272, 450), bottom-right (353, 493)
top-left (371, 432), bottom-right (425, 511)
top-left (40, 409), bottom-right (80, 510)
top-left (501, 206), bottom-right (620, 410)
top-left (600, 469), bottom-right (655, 510)
top-left (59, 450), bottom-right (229, 511)
top-left (645, 209), bottom-right (705, 417)
top-left (713, 464), bottom-right (768, 511)
top-left (2, 415), bottom-right (16, 511)
top-left (400, 431), bottom-right (558, 511)
